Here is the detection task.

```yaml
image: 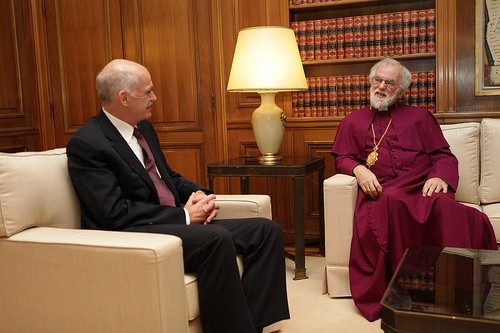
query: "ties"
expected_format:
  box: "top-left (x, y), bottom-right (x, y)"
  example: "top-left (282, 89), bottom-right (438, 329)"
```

top-left (134, 127), bottom-right (174, 208)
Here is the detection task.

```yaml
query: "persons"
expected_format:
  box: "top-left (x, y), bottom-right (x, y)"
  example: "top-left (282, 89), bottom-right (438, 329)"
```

top-left (66, 60), bottom-right (290, 333)
top-left (330, 58), bottom-right (499, 323)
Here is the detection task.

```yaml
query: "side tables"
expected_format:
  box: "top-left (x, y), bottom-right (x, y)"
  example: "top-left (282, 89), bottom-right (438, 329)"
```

top-left (208, 158), bottom-right (324, 281)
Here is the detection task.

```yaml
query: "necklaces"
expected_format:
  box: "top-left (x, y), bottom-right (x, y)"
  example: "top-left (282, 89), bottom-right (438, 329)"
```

top-left (366, 118), bottom-right (391, 167)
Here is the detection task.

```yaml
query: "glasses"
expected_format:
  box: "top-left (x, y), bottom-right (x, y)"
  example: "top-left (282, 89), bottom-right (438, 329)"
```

top-left (372, 77), bottom-right (403, 88)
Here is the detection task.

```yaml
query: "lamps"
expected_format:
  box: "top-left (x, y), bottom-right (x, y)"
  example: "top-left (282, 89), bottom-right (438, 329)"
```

top-left (227, 26), bottom-right (309, 160)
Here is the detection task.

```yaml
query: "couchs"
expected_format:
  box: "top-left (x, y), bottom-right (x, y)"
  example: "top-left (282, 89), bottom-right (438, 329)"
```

top-left (0, 146), bottom-right (271, 333)
top-left (322, 118), bottom-right (500, 298)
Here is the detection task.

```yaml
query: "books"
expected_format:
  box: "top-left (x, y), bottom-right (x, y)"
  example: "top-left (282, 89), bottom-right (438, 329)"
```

top-left (382, 9), bottom-right (436, 56)
top-left (291, 14), bottom-right (383, 61)
top-left (328, 74), bottom-right (374, 117)
top-left (401, 265), bottom-right (434, 290)
top-left (397, 69), bottom-right (435, 113)
top-left (292, 76), bottom-right (329, 117)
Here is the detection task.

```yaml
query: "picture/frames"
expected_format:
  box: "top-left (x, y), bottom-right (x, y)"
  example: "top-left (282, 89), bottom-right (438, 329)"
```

top-left (474, 0), bottom-right (500, 96)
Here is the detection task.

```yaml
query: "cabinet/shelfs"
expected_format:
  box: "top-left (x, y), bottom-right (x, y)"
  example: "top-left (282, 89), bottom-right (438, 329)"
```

top-left (286, 0), bottom-right (437, 129)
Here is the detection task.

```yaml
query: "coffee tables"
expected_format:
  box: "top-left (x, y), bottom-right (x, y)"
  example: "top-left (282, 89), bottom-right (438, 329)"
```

top-left (380, 246), bottom-right (500, 333)
